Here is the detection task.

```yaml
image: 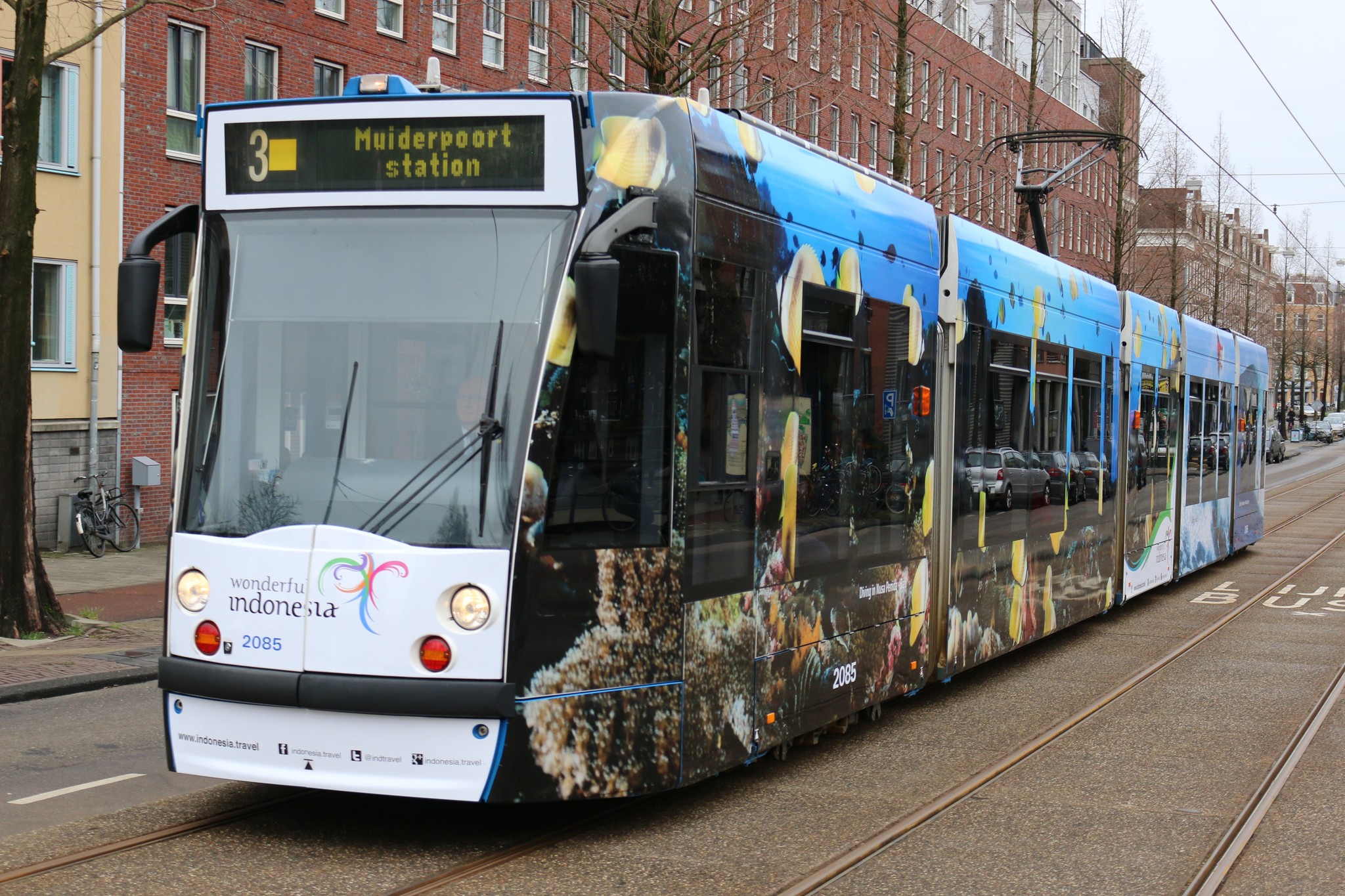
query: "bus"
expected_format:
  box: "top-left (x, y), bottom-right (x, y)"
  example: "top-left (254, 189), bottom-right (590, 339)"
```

top-left (114, 72), bottom-right (1271, 814)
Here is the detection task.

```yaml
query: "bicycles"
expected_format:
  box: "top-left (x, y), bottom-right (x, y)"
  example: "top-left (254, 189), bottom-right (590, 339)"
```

top-left (1302, 423), bottom-right (1328, 443)
top-left (792, 449), bottom-right (909, 517)
top-left (72, 471), bottom-right (141, 557)
top-left (1288, 420), bottom-right (1294, 432)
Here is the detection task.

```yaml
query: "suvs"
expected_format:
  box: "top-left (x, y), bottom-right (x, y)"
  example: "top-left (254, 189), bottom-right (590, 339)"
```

top-left (1266, 425), bottom-right (1282, 464)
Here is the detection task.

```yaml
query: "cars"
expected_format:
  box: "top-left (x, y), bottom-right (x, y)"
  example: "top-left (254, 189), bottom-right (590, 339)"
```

top-left (1288, 406), bottom-right (1321, 419)
top-left (1327, 412), bottom-right (1345, 429)
top-left (1308, 421), bottom-right (1334, 444)
top-left (895, 457), bottom-right (975, 512)
top-left (1322, 417), bottom-right (1345, 438)
top-left (1278, 432), bottom-right (1287, 460)
top-left (964, 437), bottom-right (1178, 512)
top-left (1187, 426), bottom-right (1257, 470)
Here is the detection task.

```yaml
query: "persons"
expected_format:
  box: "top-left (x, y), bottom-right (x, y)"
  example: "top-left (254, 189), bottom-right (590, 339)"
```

top-left (432, 373), bottom-right (520, 463)
top-left (1276, 408), bottom-right (1295, 429)
